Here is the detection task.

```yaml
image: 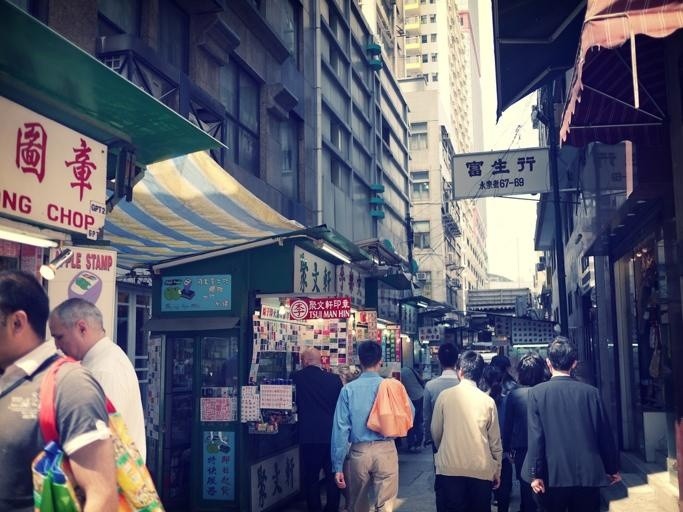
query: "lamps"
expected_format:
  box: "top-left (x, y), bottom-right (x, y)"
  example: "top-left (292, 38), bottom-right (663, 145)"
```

top-left (39, 247), bottom-right (72, 282)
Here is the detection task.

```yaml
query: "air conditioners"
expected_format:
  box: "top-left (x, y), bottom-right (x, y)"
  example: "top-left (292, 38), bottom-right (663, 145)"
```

top-left (417, 273), bottom-right (426, 281)
top-left (451, 278), bottom-right (460, 287)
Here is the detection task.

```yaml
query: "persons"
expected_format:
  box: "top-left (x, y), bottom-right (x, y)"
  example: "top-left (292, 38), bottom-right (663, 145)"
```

top-left (294, 347), bottom-right (344, 512)
top-left (0, 270), bottom-right (121, 512)
top-left (401, 335), bottom-right (622, 512)
top-left (49, 297), bottom-right (147, 467)
top-left (330, 341), bottom-right (415, 512)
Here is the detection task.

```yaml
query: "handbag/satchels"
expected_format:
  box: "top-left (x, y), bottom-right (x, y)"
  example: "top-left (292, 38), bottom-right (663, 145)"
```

top-left (30, 356), bottom-right (166, 512)
